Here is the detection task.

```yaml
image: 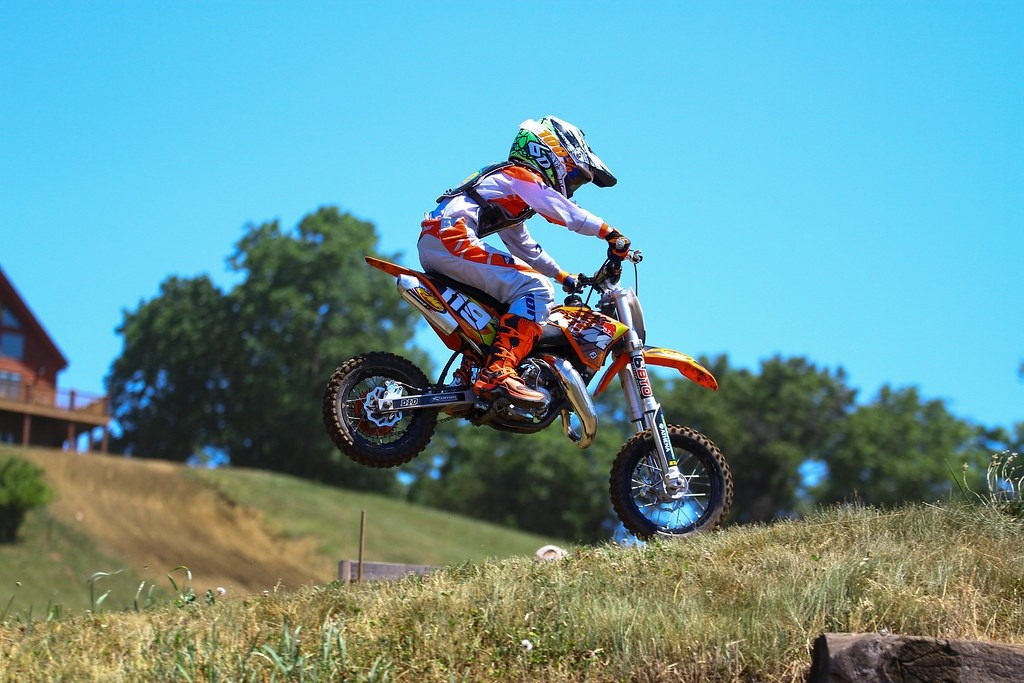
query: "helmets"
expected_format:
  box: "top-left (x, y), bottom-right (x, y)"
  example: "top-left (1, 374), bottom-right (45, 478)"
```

top-left (508, 115), bottom-right (617, 199)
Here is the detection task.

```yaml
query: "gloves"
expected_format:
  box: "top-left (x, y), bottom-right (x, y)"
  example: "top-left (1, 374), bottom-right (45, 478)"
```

top-left (562, 274), bottom-right (586, 294)
top-left (605, 228), bottom-right (631, 262)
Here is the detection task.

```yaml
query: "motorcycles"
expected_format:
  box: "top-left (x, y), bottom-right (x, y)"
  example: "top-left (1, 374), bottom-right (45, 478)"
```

top-left (321, 236), bottom-right (734, 543)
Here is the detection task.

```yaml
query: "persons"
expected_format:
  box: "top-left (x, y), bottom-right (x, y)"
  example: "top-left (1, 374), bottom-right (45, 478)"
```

top-left (416, 116), bottom-right (632, 409)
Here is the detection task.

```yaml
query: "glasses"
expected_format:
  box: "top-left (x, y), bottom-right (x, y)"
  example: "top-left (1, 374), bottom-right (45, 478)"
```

top-left (566, 168), bottom-right (588, 192)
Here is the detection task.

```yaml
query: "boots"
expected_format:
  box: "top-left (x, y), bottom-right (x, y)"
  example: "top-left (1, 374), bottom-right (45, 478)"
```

top-left (444, 349), bottom-right (484, 415)
top-left (473, 313), bottom-right (546, 409)
